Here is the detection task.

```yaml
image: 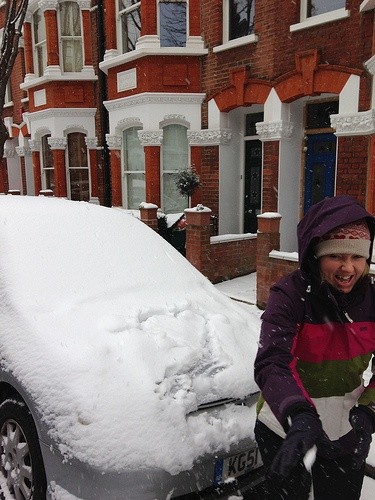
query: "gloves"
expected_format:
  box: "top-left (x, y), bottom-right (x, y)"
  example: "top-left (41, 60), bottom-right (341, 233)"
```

top-left (264, 407), bottom-right (335, 478)
top-left (323, 413), bottom-right (375, 473)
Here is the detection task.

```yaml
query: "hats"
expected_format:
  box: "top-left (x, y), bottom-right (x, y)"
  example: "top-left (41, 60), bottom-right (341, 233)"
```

top-left (312, 217), bottom-right (374, 259)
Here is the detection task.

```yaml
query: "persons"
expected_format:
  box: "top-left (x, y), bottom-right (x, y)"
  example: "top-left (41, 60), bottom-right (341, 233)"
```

top-left (253, 194), bottom-right (375, 500)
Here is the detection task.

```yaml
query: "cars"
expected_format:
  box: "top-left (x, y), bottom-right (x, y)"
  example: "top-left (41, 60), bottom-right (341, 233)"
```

top-left (1, 190), bottom-right (284, 499)
